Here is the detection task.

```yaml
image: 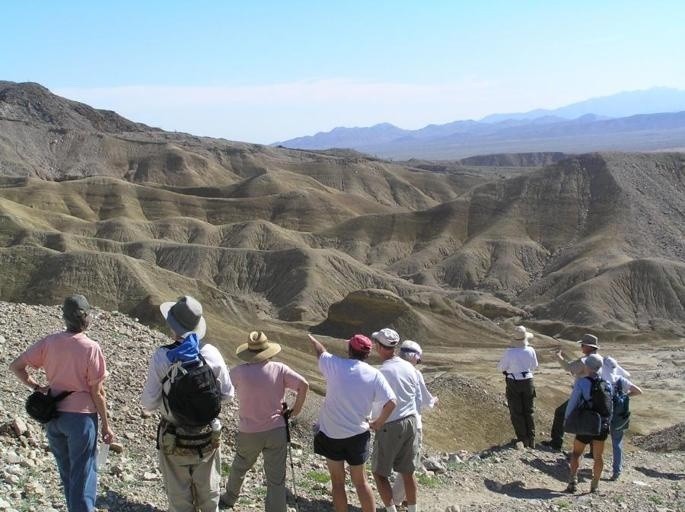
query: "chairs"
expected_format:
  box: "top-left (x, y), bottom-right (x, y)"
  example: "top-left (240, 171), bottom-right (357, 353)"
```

top-left (541, 441), bottom-right (618, 493)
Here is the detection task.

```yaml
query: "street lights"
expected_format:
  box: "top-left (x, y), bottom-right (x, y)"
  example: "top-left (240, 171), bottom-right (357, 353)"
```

top-left (26, 392), bottom-right (56, 423)
top-left (565, 410), bottom-right (601, 436)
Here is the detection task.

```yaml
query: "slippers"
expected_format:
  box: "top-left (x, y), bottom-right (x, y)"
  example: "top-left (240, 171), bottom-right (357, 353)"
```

top-left (611, 378), bottom-right (630, 432)
top-left (160, 344), bottom-right (221, 427)
top-left (584, 376), bottom-right (612, 417)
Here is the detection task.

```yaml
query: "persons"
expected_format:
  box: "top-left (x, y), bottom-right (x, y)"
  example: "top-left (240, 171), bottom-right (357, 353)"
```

top-left (9, 294), bottom-right (114, 512)
top-left (140, 295), bottom-right (235, 512)
top-left (392, 340), bottom-right (438, 512)
top-left (498, 325), bottom-right (538, 448)
top-left (307, 333), bottom-right (396, 512)
top-left (220, 329), bottom-right (308, 512)
top-left (372, 328), bottom-right (418, 512)
top-left (542, 333), bottom-right (641, 493)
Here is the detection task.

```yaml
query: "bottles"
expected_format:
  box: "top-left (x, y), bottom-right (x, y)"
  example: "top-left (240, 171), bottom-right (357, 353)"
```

top-left (511, 326), bottom-right (533, 346)
top-left (344, 334), bottom-right (373, 353)
top-left (577, 334), bottom-right (599, 349)
top-left (160, 296), bottom-right (207, 340)
top-left (581, 354), bottom-right (603, 372)
top-left (236, 331), bottom-right (281, 363)
top-left (62, 295), bottom-right (90, 322)
top-left (371, 328), bottom-right (400, 347)
